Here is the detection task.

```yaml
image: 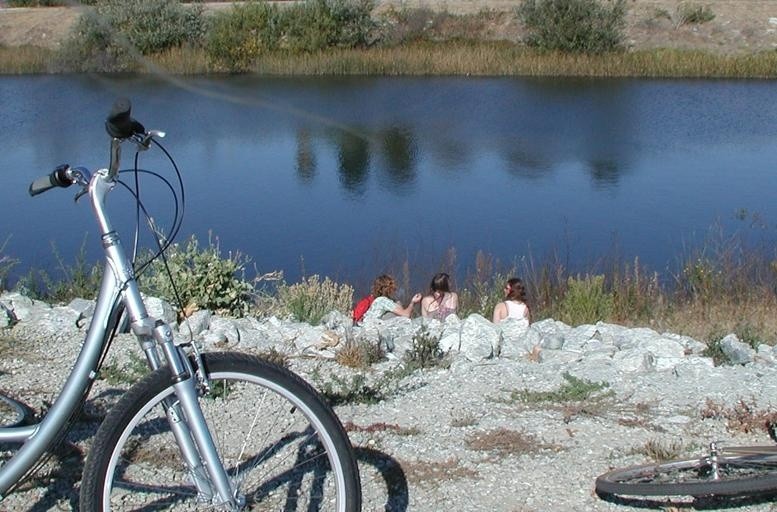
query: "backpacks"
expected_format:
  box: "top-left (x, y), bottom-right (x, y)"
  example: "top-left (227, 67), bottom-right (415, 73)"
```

top-left (352, 294), bottom-right (376, 323)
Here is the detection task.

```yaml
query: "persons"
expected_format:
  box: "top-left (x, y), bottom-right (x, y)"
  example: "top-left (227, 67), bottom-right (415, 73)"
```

top-left (356, 273), bottom-right (422, 327)
top-left (493, 278), bottom-right (532, 325)
top-left (419, 273), bottom-right (461, 323)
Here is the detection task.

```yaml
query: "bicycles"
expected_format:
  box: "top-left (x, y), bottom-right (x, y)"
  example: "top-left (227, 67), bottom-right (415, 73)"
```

top-left (0, 97), bottom-right (360, 512)
top-left (597, 419), bottom-right (777, 495)
